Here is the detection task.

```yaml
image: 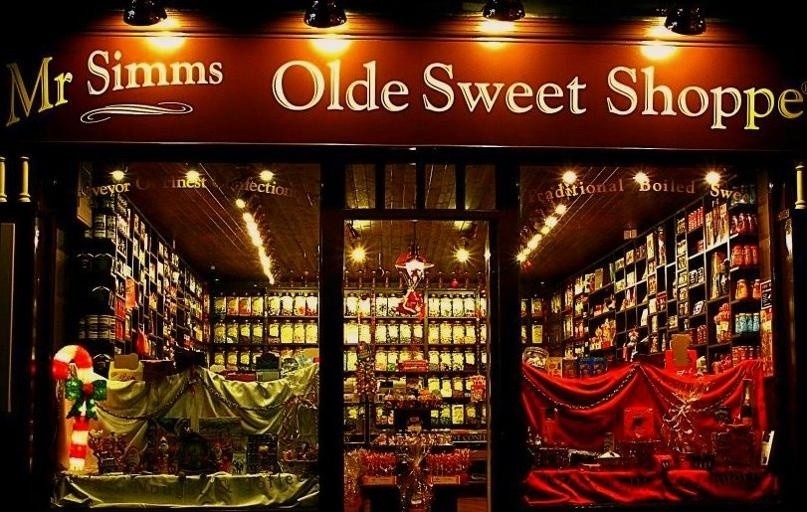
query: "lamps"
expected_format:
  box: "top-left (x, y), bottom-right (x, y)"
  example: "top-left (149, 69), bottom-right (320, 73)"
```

top-left (123, 0), bottom-right (167, 26)
top-left (111, 161), bottom-right (282, 286)
top-left (343, 221), bottom-right (479, 264)
top-left (664, 0), bottom-right (706, 35)
top-left (483, 0), bottom-right (525, 21)
top-left (303, 0), bottom-right (347, 28)
top-left (515, 166), bottom-right (724, 263)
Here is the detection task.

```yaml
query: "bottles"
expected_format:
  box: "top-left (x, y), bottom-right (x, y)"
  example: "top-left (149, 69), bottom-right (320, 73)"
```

top-left (82, 196), bottom-right (117, 245)
top-left (75, 304), bottom-right (118, 353)
top-left (687, 207), bottom-right (703, 230)
top-left (212, 270), bottom-right (543, 431)
top-left (728, 210), bottom-right (766, 332)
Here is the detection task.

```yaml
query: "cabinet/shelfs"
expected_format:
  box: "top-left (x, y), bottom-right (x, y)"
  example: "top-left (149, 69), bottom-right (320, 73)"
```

top-left (76, 188), bottom-right (209, 379)
top-left (210, 272), bottom-right (546, 448)
top-left (546, 173), bottom-right (761, 379)
